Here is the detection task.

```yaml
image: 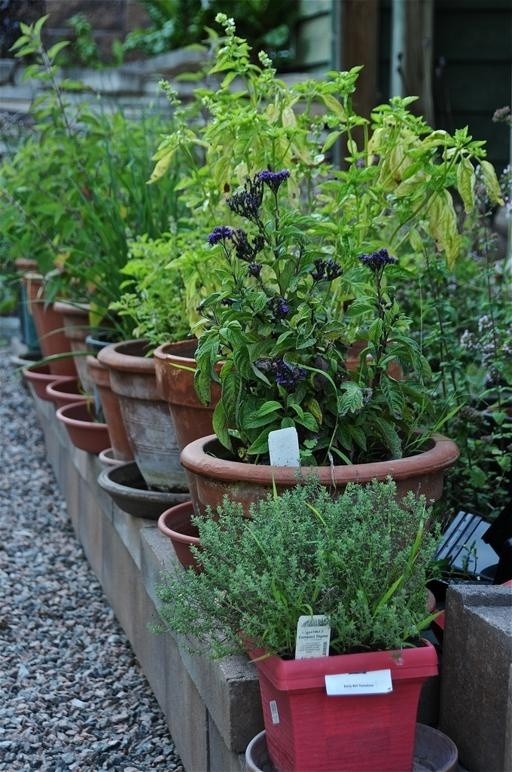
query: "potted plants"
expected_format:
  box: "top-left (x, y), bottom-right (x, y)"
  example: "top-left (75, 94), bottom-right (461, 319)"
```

top-left (0, 2), bottom-right (512, 772)
